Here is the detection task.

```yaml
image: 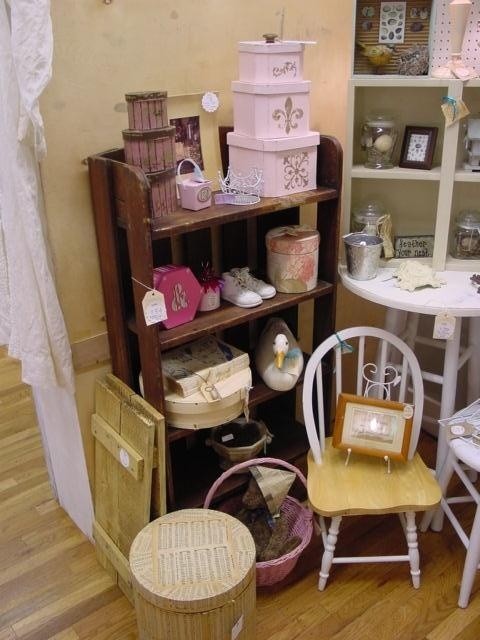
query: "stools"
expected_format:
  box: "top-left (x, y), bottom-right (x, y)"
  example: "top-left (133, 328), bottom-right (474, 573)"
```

top-left (418, 433), bottom-right (480, 609)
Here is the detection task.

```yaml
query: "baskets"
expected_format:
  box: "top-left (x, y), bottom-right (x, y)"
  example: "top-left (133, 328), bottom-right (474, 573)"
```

top-left (201, 455), bottom-right (315, 589)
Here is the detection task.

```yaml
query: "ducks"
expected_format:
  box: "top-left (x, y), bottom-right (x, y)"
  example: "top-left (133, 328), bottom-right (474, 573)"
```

top-left (253, 315), bottom-right (305, 394)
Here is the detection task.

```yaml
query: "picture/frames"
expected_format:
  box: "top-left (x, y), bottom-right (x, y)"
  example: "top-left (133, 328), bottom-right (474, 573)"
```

top-left (164, 92), bottom-right (226, 195)
top-left (332, 392), bottom-right (416, 466)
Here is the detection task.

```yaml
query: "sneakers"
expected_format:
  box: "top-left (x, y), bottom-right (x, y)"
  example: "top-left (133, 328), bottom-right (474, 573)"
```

top-left (219, 266), bottom-right (277, 309)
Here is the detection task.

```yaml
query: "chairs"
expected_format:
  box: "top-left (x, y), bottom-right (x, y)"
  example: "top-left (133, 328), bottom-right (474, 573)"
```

top-left (302, 326), bottom-right (443, 592)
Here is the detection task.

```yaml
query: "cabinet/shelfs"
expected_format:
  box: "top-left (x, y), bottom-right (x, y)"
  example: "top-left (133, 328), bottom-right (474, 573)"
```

top-left (339, 77), bottom-right (480, 272)
top-left (80, 124), bottom-right (344, 521)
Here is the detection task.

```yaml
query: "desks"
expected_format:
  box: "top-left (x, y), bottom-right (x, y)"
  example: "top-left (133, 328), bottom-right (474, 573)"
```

top-left (336, 264), bottom-right (480, 533)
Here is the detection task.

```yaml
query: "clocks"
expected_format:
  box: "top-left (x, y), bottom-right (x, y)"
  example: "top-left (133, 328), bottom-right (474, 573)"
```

top-left (398, 124), bottom-right (439, 171)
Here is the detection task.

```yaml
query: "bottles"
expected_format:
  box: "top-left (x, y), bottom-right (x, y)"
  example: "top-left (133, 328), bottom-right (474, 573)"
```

top-left (358, 112), bottom-right (400, 170)
top-left (350, 203), bottom-right (384, 257)
top-left (448, 210), bottom-right (480, 260)
